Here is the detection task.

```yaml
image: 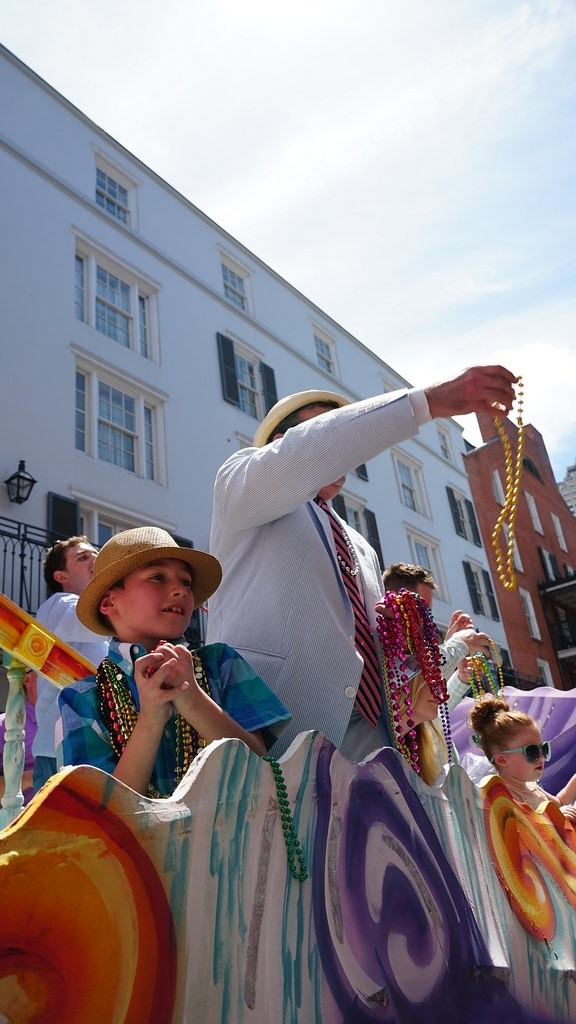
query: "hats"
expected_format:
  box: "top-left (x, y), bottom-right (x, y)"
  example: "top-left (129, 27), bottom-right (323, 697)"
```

top-left (76, 526), bottom-right (222, 636)
top-left (253, 390), bottom-right (352, 448)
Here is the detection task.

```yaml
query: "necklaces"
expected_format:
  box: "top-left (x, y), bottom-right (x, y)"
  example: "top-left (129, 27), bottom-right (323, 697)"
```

top-left (492, 375), bottom-right (524, 591)
top-left (469, 638), bottom-right (505, 744)
top-left (376, 588), bottom-right (452, 780)
top-left (336, 524), bottom-right (361, 576)
top-left (262, 755), bottom-right (308, 881)
top-left (96, 648), bottom-right (210, 798)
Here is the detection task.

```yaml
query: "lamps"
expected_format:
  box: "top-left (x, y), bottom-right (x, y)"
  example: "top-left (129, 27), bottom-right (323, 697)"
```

top-left (3, 461), bottom-right (37, 504)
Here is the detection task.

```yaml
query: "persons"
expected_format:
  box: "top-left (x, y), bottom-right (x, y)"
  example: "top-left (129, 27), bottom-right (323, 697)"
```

top-left (204, 364), bottom-right (519, 761)
top-left (472, 700), bottom-right (576, 821)
top-left (57, 527), bottom-right (292, 801)
top-left (0, 668), bottom-right (38, 807)
top-left (31, 537), bottom-right (114, 796)
top-left (396, 674), bottom-right (447, 786)
top-left (383, 563), bottom-right (490, 764)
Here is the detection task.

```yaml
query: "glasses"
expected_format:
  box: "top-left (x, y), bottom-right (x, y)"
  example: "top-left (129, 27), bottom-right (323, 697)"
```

top-left (489, 741), bottom-right (551, 764)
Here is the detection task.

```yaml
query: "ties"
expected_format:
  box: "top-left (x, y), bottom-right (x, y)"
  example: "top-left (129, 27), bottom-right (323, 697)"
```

top-left (314, 497), bottom-right (382, 727)
